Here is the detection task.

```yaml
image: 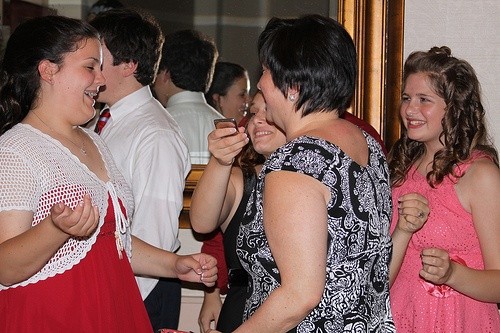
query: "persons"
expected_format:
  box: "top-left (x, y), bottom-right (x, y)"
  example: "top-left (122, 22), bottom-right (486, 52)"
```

top-left (0, 18), bottom-right (217, 333)
top-left (189, 92), bottom-right (285, 333)
top-left (230, 15), bottom-right (395, 333)
top-left (154, 30), bottom-right (251, 164)
top-left (198, 109), bottom-right (383, 333)
top-left (386, 47), bottom-right (500, 333)
top-left (90, 9), bottom-right (190, 333)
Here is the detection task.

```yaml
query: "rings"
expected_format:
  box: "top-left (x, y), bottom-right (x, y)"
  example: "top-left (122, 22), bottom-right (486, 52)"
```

top-left (418, 212), bottom-right (425, 219)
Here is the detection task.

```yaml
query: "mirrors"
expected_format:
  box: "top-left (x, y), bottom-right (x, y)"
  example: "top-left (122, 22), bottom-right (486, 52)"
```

top-left (44, 0), bottom-right (404, 233)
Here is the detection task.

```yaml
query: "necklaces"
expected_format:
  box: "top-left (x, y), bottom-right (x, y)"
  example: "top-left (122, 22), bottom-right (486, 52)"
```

top-left (30, 110), bottom-right (87, 157)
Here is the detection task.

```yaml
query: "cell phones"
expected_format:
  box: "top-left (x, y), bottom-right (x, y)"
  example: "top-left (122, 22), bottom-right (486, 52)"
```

top-left (213, 117), bottom-right (239, 139)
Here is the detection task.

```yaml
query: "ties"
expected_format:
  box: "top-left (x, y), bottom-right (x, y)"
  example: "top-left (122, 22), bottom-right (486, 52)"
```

top-left (94, 109), bottom-right (111, 134)
